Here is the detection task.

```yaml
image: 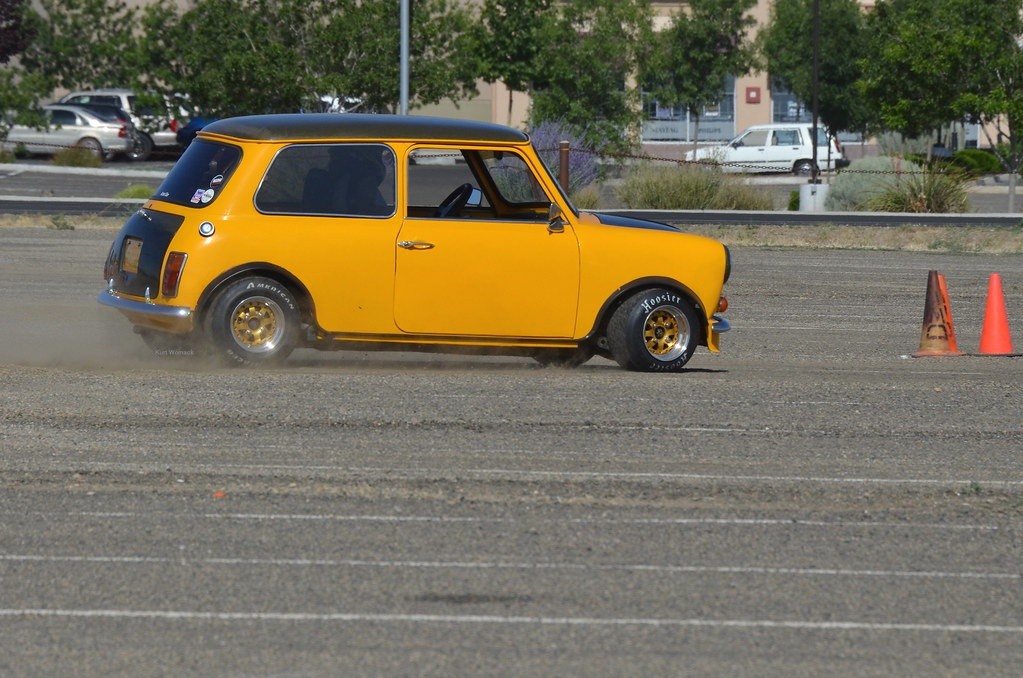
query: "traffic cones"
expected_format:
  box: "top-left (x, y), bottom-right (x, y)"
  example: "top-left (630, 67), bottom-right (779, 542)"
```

top-left (971, 273), bottom-right (1023, 358)
top-left (937, 273), bottom-right (966, 356)
top-left (911, 268), bottom-right (957, 357)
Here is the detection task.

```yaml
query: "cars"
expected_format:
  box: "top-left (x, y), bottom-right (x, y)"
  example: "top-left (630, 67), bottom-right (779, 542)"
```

top-left (94, 112), bottom-right (732, 375)
top-left (0, 91), bottom-right (222, 161)
top-left (684, 123), bottom-right (850, 176)
